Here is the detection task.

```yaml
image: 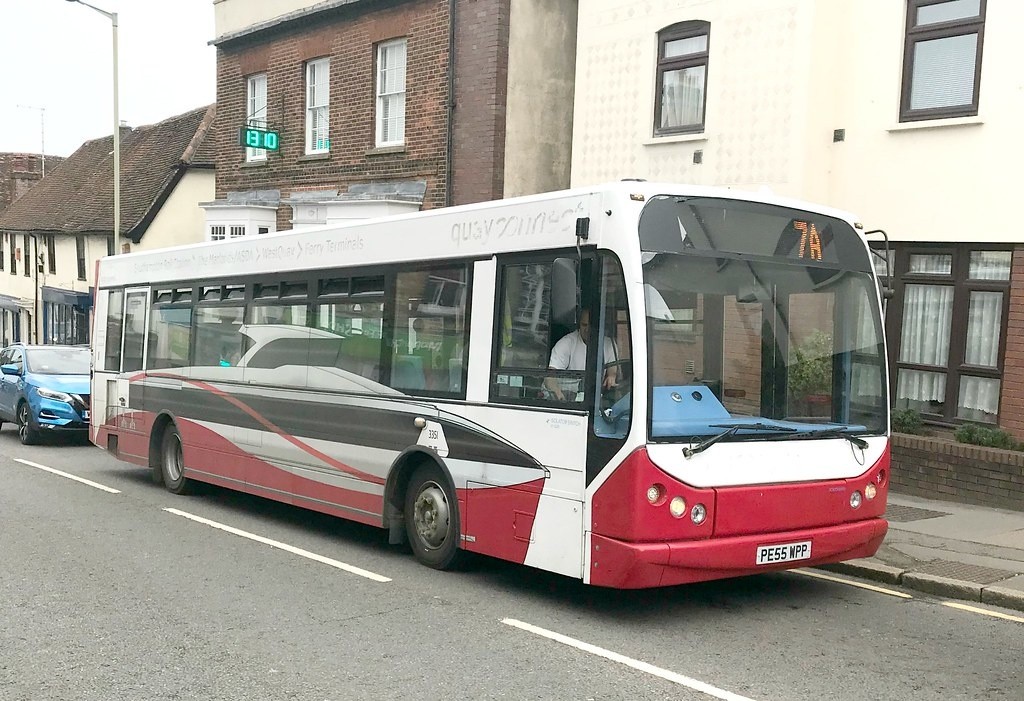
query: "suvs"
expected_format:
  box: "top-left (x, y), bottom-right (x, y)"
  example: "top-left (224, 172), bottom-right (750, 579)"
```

top-left (0, 341), bottom-right (94, 446)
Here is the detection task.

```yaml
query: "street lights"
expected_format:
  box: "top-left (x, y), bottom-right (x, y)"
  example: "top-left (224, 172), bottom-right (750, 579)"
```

top-left (65, 0), bottom-right (120, 256)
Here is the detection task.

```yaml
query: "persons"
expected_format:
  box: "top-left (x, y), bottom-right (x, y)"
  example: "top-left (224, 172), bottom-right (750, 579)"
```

top-left (540, 309), bottom-right (618, 401)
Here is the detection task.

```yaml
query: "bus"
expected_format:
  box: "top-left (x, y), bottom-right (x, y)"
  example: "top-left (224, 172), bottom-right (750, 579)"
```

top-left (84, 178), bottom-right (896, 595)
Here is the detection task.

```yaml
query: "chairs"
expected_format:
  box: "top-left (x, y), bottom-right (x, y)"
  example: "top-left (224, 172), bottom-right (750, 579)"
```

top-left (395, 355), bottom-right (428, 391)
